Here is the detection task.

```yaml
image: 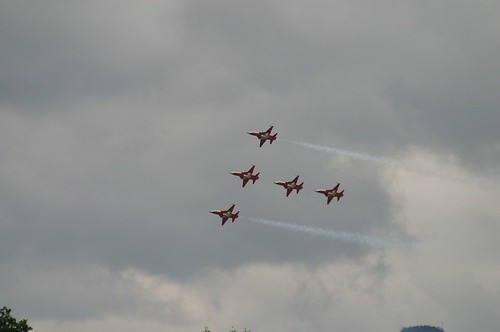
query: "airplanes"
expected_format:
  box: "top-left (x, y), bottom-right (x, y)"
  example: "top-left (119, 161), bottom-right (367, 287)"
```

top-left (207, 126), bottom-right (344, 229)
